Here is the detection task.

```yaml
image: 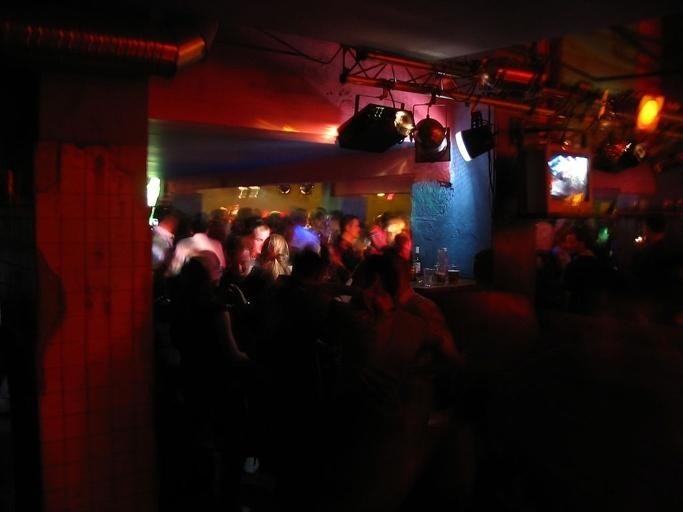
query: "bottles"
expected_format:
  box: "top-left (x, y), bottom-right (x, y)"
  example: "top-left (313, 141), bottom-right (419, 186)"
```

top-left (413, 244), bottom-right (423, 276)
top-left (437, 248), bottom-right (449, 278)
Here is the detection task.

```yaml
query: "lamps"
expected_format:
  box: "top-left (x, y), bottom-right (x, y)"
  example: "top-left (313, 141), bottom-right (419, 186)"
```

top-left (338, 45), bottom-right (495, 163)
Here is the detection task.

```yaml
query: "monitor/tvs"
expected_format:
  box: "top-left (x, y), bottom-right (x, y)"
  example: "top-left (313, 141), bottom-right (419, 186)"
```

top-left (525, 143), bottom-right (593, 216)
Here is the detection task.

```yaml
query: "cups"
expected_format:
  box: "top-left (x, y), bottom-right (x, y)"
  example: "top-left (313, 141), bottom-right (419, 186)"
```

top-left (424, 266), bottom-right (437, 287)
top-left (448, 269), bottom-right (462, 282)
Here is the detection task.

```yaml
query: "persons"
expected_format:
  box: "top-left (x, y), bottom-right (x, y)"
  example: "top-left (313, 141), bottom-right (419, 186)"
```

top-left (151, 195), bottom-right (682, 512)
